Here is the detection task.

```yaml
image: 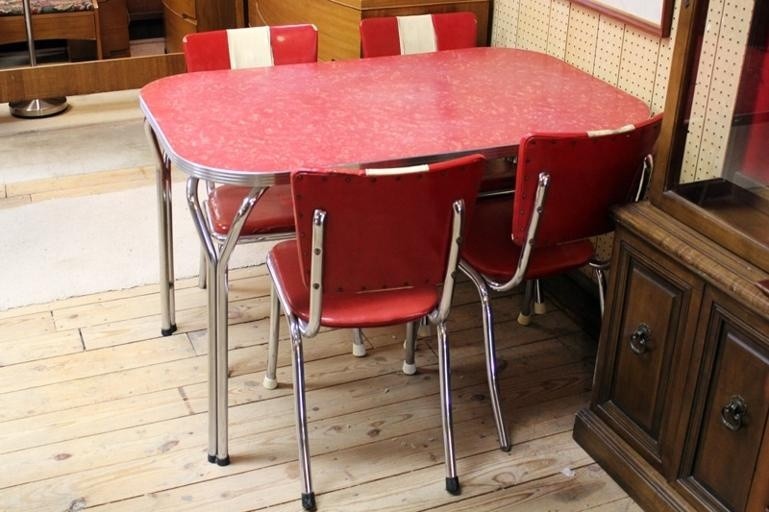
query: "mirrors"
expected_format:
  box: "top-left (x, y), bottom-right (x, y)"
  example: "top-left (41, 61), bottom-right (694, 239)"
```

top-left (652, 0), bottom-right (768, 271)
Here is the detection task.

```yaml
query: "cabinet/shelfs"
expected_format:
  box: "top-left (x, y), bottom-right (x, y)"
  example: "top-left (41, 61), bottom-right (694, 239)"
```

top-left (160, 0), bottom-right (243, 53)
top-left (575, 205), bottom-right (768, 511)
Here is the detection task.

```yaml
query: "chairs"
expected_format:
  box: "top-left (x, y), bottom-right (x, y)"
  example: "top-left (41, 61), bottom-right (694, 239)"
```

top-left (419, 114), bottom-right (669, 459)
top-left (261, 150), bottom-right (487, 512)
top-left (358, 8), bottom-right (549, 327)
top-left (181, 20), bottom-right (370, 381)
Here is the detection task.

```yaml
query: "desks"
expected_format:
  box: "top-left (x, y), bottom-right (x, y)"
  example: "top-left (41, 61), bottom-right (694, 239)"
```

top-left (135, 38), bottom-right (661, 471)
top-left (727, 50), bottom-right (769, 185)
